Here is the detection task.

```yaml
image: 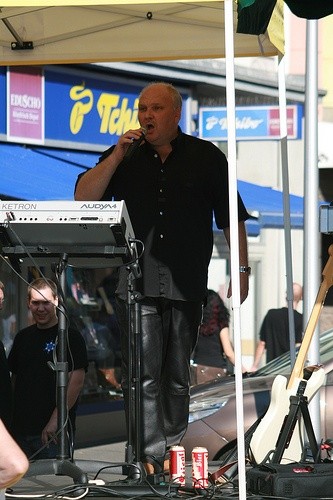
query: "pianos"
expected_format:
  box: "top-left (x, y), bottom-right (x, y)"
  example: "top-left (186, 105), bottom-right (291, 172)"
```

top-left (0, 197), bottom-right (144, 483)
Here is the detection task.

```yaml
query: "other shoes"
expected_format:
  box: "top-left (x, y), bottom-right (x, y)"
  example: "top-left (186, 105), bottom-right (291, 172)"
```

top-left (164, 459), bottom-right (170, 471)
top-left (144, 463), bottom-right (154, 475)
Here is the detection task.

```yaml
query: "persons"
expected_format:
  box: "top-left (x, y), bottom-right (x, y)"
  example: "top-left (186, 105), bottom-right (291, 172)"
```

top-left (252, 283), bottom-right (303, 373)
top-left (0, 280), bottom-right (123, 493)
top-left (192, 290), bottom-right (235, 385)
top-left (75, 83), bottom-right (250, 481)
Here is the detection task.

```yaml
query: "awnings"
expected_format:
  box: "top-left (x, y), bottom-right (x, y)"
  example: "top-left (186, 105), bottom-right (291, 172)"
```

top-left (0, 145), bottom-right (303, 236)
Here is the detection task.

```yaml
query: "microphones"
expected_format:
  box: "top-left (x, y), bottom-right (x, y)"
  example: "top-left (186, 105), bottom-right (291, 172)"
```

top-left (128, 128), bottom-right (147, 149)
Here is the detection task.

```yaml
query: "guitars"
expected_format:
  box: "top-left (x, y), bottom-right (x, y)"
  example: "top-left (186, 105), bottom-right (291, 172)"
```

top-left (245, 245), bottom-right (333, 465)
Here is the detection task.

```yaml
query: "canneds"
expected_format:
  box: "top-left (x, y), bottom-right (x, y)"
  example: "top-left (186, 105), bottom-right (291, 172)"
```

top-left (169, 445), bottom-right (185, 486)
top-left (191, 447), bottom-right (209, 489)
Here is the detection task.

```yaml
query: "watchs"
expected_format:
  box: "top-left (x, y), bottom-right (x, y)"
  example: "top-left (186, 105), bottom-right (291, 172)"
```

top-left (239, 266), bottom-right (251, 274)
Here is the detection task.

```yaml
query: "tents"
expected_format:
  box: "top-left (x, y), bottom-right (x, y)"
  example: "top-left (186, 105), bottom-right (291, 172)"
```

top-left (0, 0), bottom-right (296, 500)
